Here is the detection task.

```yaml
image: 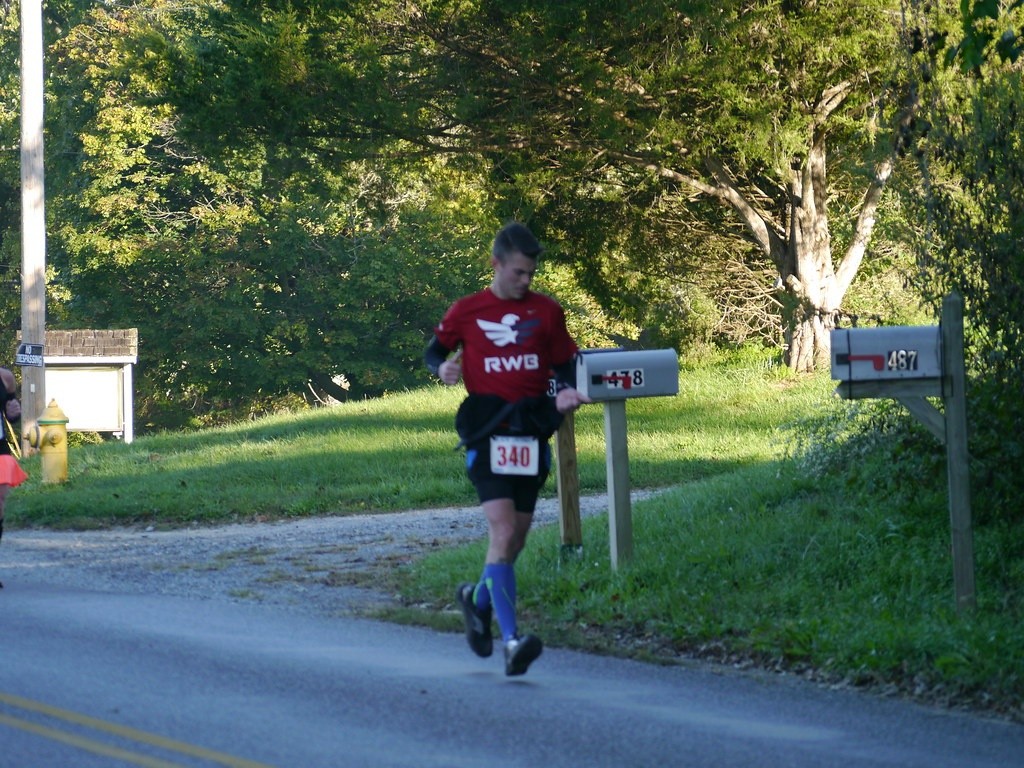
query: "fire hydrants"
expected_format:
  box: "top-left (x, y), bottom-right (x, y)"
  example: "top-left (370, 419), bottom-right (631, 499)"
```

top-left (21, 398), bottom-right (70, 486)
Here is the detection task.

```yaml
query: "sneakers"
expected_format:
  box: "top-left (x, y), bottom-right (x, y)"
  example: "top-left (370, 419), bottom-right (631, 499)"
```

top-left (456, 584), bottom-right (494, 657)
top-left (504, 634), bottom-right (542, 675)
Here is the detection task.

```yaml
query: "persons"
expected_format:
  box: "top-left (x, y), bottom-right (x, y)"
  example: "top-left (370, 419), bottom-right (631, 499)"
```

top-left (0, 368), bottom-right (28, 588)
top-left (425, 222), bottom-right (591, 677)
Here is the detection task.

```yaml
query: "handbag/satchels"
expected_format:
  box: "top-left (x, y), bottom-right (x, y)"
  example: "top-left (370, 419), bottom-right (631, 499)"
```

top-left (511, 395), bottom-right (563, 443)
top-left (454, 392), bottom-right (511, 452)
